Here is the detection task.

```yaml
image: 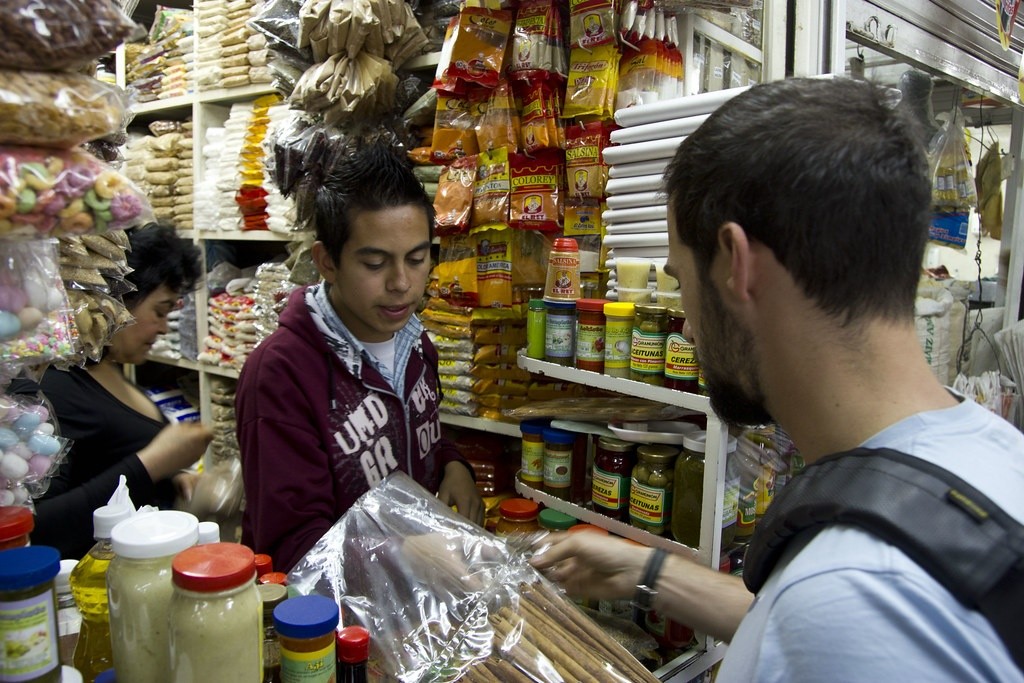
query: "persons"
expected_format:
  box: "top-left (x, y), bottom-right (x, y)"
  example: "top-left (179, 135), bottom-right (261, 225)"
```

top-left (7, 217), bottom-right (217, 560)
top-left (234, 140), bottom-right (486, 575)
top-left (529, 73), bottom-right (1024, 682)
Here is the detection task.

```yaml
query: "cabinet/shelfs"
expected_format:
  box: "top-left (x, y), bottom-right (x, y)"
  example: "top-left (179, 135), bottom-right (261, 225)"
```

top-left (108, 0), bottom-right (1024, 683)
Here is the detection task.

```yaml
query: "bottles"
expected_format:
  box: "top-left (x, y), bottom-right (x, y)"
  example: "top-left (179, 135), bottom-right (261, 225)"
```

top-left (574, 596), bottom-right (694, 647)
top-left (0, 506), bottom-right (34, 549)
top-left (255, 554), bottom-right (370, 683)
top-left (495, 499), bottom-right (649, 548)
top-left (519, 420), bottom-right (574, 488)
top-left (69, 504), bottom-right (132, 683)
top-left (664, 307), bottom-right (710, 390)
top-left (591, 437), bottom-right (680, 528)
top-left (631, 305), bottom-right (667, 375)
top-left (0, 547), bottom-right (85, 683)
top-left (735, 424), bottom-right (807, 537)
top-left (527, 299), bottom-right (544, 359)
top-left (720, 553), bottom-right (748, 578)
top-left (106, 510), bottom-right (200, 683)
top-left (543, 300), bottom-right (575, 357)
top-left (544, 238), bottom-right (582, 300)
top-left (672, 431), bottom-right (740, 552)
top-left (165, 521), bottom-right (263, 683)
top-left (432, 276), bottom-right (545, 308)
top-left (604, 302), bottom-right (635, 368)
top-left (575, 299), bottom-right (613, 366)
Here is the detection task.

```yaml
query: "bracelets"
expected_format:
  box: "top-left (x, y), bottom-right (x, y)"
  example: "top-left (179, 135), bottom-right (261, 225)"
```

top-left (630, 545), bottom-right (671, 613)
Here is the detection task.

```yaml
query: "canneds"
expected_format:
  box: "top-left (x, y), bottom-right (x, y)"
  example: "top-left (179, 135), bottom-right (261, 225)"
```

top-left (495, 299), bottom-right (777, 646)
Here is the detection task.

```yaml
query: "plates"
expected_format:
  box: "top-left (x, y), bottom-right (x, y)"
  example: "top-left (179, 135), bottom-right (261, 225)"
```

top-left (608, 420), bottom-right (701, 444)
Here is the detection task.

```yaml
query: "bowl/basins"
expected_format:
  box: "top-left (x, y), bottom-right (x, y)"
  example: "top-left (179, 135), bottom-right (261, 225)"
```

top-left (615, 258), bottom-right (682, 311)
